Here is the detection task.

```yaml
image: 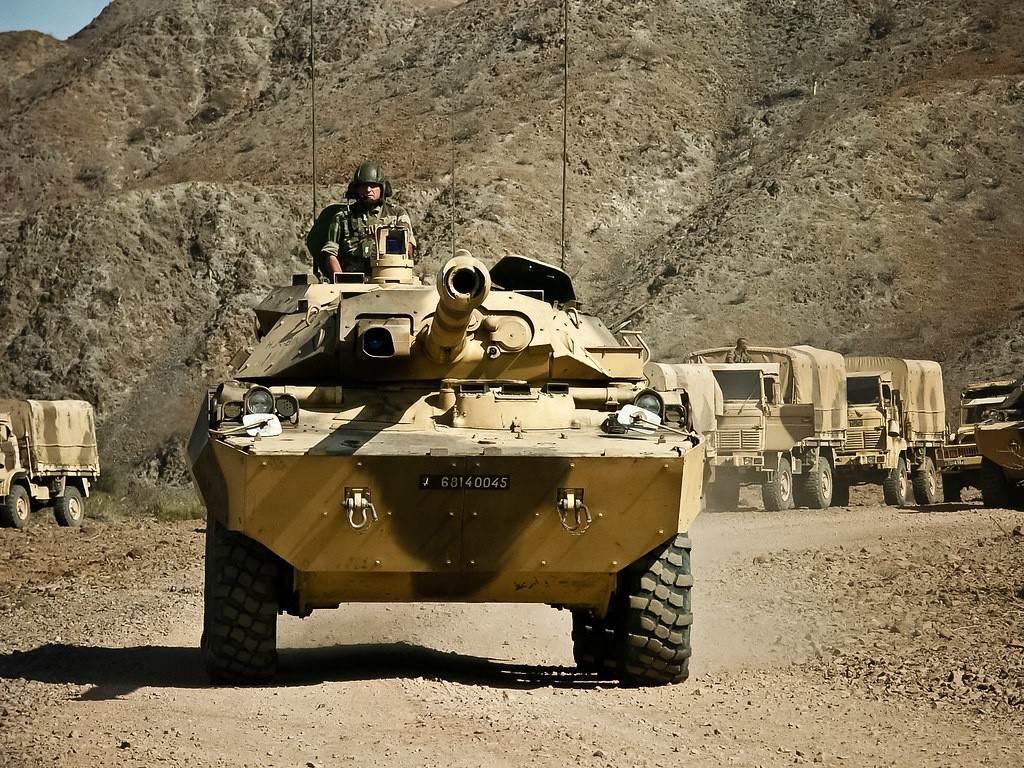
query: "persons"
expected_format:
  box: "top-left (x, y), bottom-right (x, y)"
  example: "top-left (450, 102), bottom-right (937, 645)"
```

top-left (725, 338), bottom-right (751, 363)
top-left (320, 162), bottom-right (417, 275)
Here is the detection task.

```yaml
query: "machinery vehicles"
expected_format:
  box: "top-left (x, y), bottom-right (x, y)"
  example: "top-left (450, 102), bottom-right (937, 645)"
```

top-left (175, 227), bottom-right (695, 689)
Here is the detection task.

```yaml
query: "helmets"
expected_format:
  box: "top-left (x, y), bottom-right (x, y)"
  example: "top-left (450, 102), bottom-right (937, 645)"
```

top-left (344, 163), bottom-right (392, 203)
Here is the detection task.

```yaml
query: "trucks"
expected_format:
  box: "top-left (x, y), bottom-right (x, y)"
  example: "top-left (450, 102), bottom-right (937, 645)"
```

top-left (671, 363), bottom-right (724, 499)
top-left (684, 345), bottom-right (848, 512)
top-left (937, 376), bottom-right (1023, 510)
top-left (831, 356), bottom-right (946, 508)
top-left (0, 398), bottom-right (100, 529)
